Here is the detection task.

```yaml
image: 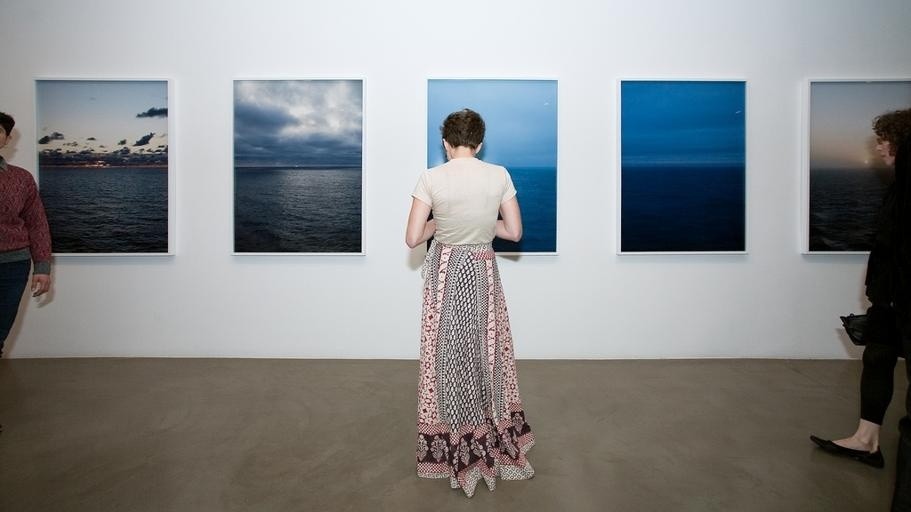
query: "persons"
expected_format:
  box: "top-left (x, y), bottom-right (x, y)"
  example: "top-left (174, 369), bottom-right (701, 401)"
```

top-left (809, 107), bottom-right (911, 468)
top-left (0, 113), bottom-right (53, 361)
top-left (405, 109), bottom-right (536, 499)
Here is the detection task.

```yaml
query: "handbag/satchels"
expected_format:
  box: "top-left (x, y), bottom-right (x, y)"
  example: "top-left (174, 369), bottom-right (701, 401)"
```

top-left (839, 313), bottom-right (868, 345)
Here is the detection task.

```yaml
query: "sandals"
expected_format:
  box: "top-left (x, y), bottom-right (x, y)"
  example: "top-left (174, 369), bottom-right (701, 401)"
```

top-left (810, 435), bottom-right (885, 468)
